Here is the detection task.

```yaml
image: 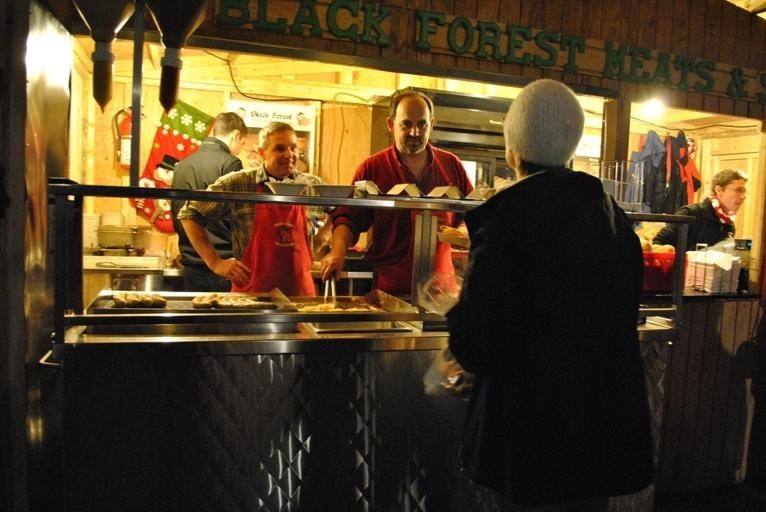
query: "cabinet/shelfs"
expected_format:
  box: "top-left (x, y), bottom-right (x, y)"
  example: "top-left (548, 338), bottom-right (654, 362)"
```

top-left (38, 174), bottom-right (698, 509)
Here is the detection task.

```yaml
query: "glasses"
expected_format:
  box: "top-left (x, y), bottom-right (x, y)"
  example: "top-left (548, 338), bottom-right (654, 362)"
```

top-left (735, 170), bottom-right (747, 180)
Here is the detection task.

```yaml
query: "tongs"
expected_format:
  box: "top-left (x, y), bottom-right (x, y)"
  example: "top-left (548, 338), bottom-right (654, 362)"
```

top-left (322, 269), bottom-right (337, 308)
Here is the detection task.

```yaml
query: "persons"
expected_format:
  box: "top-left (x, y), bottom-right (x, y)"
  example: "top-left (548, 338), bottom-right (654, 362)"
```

top-left (320, 89), bottom-right (473, 297)
top-left (446, 78), bottom-right (656, 512)
top-left (172, 112), bottom-right (248, 291)
top-left (653, 169), bottom-right (747, 289)
top-left (177, 122), bottom-right (336, 296)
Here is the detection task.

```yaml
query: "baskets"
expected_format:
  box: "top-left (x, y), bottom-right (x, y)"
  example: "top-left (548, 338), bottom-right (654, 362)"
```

top-left (642, 251), bottom-right (687, 292)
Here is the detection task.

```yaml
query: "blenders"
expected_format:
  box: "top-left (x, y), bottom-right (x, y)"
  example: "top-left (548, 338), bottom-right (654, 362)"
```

top-left (734, 239), bottom-right (753, 293)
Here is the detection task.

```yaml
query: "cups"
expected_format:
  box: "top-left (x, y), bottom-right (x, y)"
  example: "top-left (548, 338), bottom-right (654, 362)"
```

top-left (587, 160), bottom-right (643, 211)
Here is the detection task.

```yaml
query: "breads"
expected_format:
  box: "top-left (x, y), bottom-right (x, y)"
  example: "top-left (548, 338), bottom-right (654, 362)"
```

top-left (438, 223), bottom-right (470, 241)
top-left (189, 291), bottom-right (279, 312)
top-left (110, 289), bottom-right (168, 310)
top-left (297, 299), bottom-right (386, 314)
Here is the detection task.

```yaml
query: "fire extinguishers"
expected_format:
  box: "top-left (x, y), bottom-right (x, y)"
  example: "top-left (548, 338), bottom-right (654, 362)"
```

top-left (116, 106), bottom-right (144, 170)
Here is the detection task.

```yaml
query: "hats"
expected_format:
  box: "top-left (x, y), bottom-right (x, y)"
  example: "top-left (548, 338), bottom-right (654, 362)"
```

top-left (712, 169), bottom-right (748, 191)
top-left (502, 79), bottom-right (584, 168)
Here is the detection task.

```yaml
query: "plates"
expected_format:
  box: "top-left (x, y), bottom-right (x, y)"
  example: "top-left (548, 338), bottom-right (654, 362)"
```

top-left (264, 182), bottom-right (309, 196)
top-left (312, 183), bottom-right (357, 197)
